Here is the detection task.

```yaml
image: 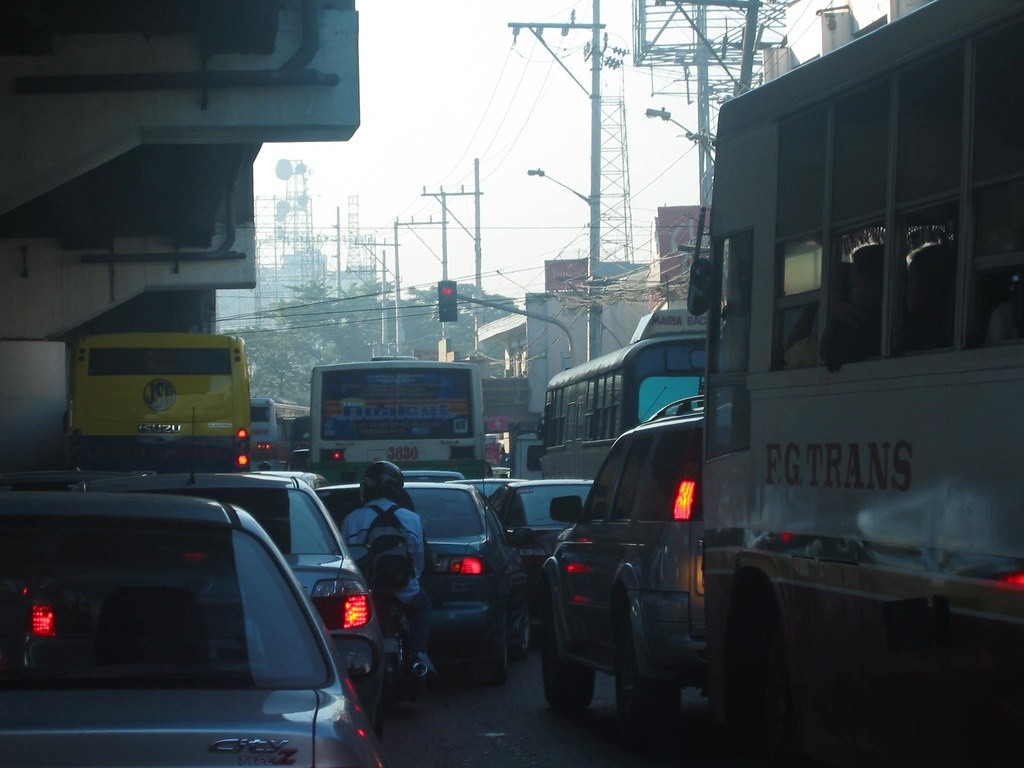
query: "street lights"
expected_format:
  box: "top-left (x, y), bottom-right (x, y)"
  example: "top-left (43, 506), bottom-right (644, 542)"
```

top-left (526, 168), bottom-right (601, 361)
top-left (642, 106), bottom-right (706, 205)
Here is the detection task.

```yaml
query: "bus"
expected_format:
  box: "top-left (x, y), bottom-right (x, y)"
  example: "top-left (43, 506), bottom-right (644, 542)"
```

top-left (65, 331), bottom-right (252, 473)
top-left (526, 299), bottom-right (708, 483)
top-left (310, 356), bottom-right (485, 485)
top-left (250, 395), bottom-right (312, 470)
top-left (687, 1), bottom-right (1023, 767)
top-left (510, 433), bottom-right (544, 480)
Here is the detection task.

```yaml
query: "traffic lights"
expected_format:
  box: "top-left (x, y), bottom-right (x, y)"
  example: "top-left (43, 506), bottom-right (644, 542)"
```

top-left (438, 280), bottom-right (458, 323)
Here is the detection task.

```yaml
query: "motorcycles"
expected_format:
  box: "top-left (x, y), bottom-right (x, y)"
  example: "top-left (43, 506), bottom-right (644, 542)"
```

top-left (370, 584), bottom-right (429, 693)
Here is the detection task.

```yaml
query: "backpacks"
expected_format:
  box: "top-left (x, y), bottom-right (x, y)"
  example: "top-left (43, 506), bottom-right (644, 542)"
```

top-left (363, 504), bottom-right (415, 589)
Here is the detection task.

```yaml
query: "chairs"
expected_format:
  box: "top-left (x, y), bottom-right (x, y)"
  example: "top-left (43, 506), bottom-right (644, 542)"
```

top-left (908, 245), bottom-right (957, 353)
top-left (853, 242), bottom-right (910, 353)
top-left (93, 571), bottom-right (209, 667)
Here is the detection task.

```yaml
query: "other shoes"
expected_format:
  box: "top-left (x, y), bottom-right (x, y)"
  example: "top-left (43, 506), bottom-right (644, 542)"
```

top-left (409, 650), bottom-right (439, 680)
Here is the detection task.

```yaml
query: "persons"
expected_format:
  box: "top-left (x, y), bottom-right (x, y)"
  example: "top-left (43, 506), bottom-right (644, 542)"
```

top-left (341, 462), bottom-right (432, 668)
top-left (783, 247), bottom-right (1024, 364)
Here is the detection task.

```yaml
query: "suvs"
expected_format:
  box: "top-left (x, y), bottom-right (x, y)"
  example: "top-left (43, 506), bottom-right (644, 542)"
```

top-left (532, 392), bottom-right (709, 716)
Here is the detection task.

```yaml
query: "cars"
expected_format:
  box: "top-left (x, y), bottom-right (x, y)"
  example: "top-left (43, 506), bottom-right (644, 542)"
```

top-left (0, 466), bottom-right (595, 768)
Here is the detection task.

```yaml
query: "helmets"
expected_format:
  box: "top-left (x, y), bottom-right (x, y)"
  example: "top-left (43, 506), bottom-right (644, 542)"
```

top-left (361, 460), bottom-right (404, 494)
